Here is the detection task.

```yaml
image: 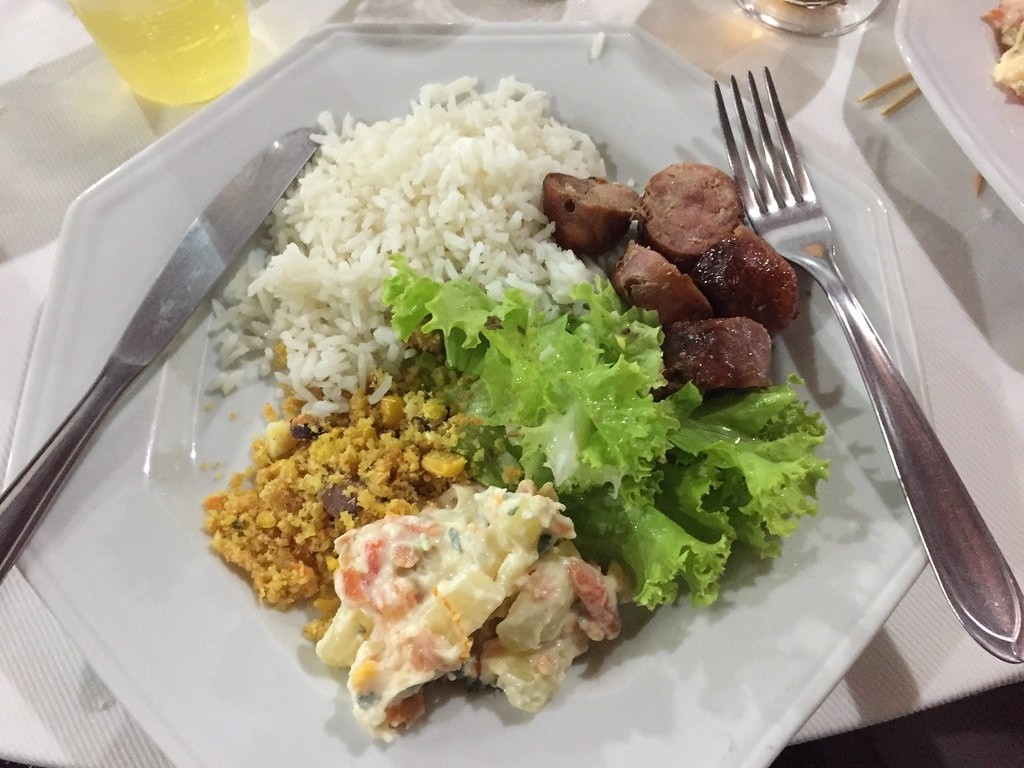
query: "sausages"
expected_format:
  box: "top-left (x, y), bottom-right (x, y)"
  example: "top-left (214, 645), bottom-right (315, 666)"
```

top-left (543, 162), bottom-right (801, 392)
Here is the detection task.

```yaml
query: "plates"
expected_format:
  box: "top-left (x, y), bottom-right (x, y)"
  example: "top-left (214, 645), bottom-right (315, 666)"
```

top-left (0, 2), bottom-right (1023, 768)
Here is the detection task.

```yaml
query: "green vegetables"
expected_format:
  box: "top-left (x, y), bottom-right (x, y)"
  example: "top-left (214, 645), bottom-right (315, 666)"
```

top-left (384, 251), bottom-right (834, 612)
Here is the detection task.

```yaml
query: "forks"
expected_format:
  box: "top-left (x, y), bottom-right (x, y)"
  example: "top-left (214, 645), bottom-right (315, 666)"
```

top-left (712, 66), bottom-right (1023, 667)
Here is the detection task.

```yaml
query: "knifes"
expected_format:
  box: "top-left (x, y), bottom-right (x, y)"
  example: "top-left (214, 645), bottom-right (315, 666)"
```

top-left (0, 123), bottom-right (322, 598)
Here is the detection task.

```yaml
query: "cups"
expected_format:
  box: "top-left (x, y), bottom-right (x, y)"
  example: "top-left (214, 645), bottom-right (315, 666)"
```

top-left (64, 1), bottom-right (253, 106)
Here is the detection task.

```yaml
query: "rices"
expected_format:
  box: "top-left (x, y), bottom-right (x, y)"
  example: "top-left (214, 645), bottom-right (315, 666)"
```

top-left (205, 72), bottom-right (612, 415)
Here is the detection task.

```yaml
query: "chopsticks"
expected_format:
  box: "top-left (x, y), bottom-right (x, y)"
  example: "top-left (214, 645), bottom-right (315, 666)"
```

top-left (854, 74), bottom-right (920, 118)
